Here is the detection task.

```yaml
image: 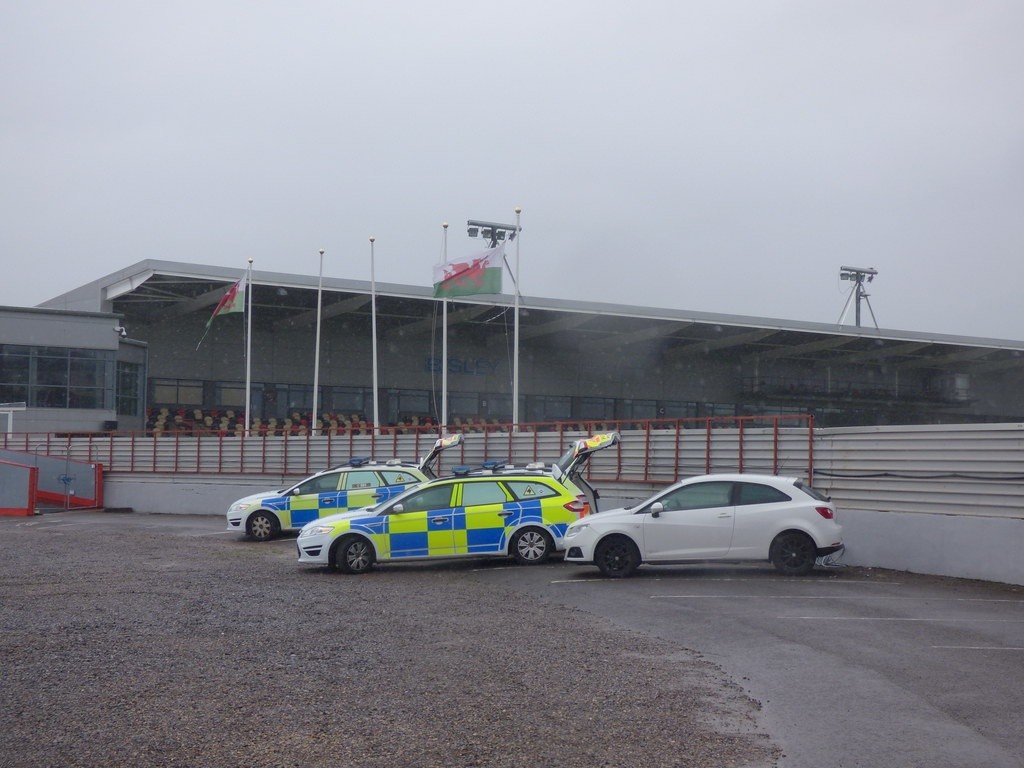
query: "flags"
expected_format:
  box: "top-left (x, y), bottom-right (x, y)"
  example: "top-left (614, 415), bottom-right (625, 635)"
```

top-left (433, 240), bottom-right (505, 300)
top-left (206, 270), bottom-right (245, 326)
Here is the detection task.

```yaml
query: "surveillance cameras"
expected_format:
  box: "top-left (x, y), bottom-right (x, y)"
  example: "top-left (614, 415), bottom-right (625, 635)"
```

top-left (121, 332), bottom-right (127, 338)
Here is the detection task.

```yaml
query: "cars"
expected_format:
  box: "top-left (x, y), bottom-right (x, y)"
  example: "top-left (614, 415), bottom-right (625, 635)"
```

top-left (224, 434), bottom-right (622, 576)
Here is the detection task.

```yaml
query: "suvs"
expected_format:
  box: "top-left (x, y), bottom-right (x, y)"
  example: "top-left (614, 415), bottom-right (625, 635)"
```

top-left (562, 472), bottom-right (845, 580)
